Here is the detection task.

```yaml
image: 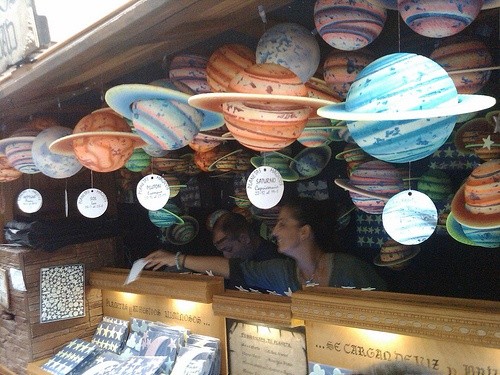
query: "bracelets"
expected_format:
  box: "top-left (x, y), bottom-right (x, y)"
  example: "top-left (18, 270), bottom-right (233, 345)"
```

top-left (176, 252), bottom-right (190, 271)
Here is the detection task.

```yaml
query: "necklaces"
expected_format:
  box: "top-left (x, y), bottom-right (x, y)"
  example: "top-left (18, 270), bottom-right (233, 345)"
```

top-left (301, 249), bottom-right (323, 288)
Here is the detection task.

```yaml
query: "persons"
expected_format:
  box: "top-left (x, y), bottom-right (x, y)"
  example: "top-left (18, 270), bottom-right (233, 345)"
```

top-left (212, 213), bottom-right (289, 293)
top-left (144, 197), bottom-right (393, 296)
